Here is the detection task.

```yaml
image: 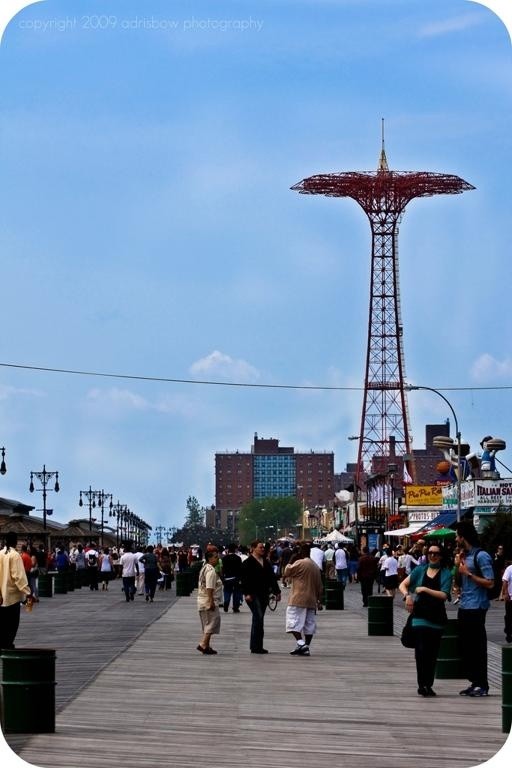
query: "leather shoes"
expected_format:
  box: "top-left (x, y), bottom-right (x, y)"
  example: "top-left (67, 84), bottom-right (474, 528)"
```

top-left (197, 645), bottom-right (214, 654)
top-left (211, 648), bottom-right (217, 654)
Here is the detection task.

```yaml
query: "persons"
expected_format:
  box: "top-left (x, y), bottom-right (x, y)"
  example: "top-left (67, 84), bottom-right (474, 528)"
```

top-left (197, 544), bottom-right (322, 655)
top-left (120, 543), bottom-right (347, 610)
top-left (21, 544), bottom-right (123, 611)
top-left (358, 535), bottom-right (512, 699)
top-left (1, 534), bottom-right (36, 650)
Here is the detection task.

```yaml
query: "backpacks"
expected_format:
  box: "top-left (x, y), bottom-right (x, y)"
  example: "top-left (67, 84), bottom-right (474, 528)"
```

top-left (474, 548), bottom-right (503, 600)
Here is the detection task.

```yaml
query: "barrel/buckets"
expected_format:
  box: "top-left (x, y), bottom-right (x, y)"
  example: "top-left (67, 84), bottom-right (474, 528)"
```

top-left (176, 562), bottom-right (203, 597)
top-left (325, 581), bottom-right (344, 611)
top-left (55, 573), bottom-right (67, 594)
top-left (501, 643), bottom-right (512, 734)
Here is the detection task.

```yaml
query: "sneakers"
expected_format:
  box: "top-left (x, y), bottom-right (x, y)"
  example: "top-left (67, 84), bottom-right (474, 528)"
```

top-left (470, 686), bottom-right (489, 697)
top-left (460, 685), bottom-right (474, 696)
top-left (290, 643), bottom-right (310, 654)
top-left (304, 648), bottom-right (310, 656)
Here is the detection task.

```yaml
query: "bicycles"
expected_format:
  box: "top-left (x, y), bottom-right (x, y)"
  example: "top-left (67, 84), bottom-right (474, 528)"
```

top-left (267, 588), bottom-right (278, 612)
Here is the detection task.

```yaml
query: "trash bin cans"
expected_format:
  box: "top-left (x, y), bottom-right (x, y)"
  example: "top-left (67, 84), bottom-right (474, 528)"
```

top-left (502, 646), bottom-right (511, 731)
top-left (54, 573), bottom-right (66, 594)
top-left (326, 582), bottom-right (343, 609)
top-left (320, 578), bottom-right (336, 606)
top-left (176, 571), bottom-right (193, 596)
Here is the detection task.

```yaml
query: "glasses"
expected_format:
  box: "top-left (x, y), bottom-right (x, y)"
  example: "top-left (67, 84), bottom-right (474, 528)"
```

top-left (427, 551), bottom-right (441, 557)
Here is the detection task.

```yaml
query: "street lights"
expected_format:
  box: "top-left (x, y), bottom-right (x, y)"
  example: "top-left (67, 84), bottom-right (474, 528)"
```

top-left (79, 484), bottom-right (179, 556)
top-left (403, 385), bottom-right (463, 548)
top-left (347, 435), bottom-right (392, 549)
top-left (245, 507), bottom-right (280, 541)
top-left (1, 446), bottom-right (7, 475)
top-left (296, 483), bottom-right (329, 540)
top-left (30, 463), bottom-right (60, 598)
top-left (228, 510), bottom-right (241, 542)
